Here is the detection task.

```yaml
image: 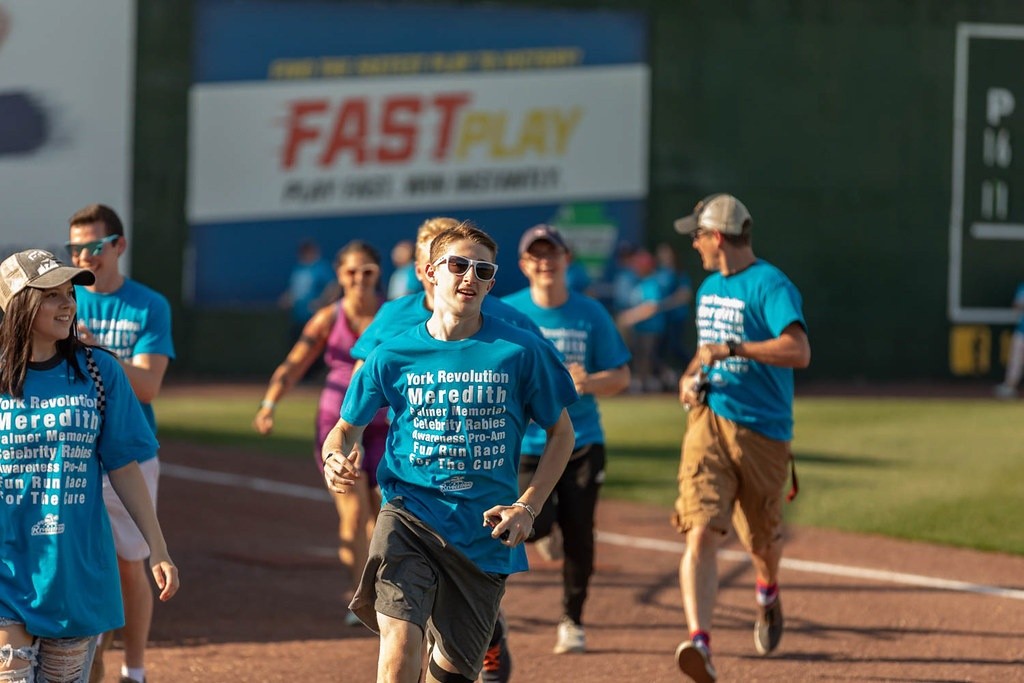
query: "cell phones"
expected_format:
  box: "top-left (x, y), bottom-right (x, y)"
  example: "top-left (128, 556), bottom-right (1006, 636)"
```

top-left (485, 515), bottom-right (535, 539)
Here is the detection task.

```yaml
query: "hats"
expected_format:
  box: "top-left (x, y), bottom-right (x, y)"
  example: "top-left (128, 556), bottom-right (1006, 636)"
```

top-left (0, 249), bottom-right (96, 313)
top-left (675, 192), bottom-right (752, 235)
top-left (519, 224), bottom-right (569, 255)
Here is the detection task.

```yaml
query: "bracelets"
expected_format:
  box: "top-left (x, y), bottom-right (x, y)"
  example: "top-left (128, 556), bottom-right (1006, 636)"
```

top-left (260, 401), bottom-right (278, 410)
top-left (322, 451), bottom-right (344, 467)
top-left (511, 501), bottom-right (536, 523)
top-left (726, 340), bottom-right (736, 356)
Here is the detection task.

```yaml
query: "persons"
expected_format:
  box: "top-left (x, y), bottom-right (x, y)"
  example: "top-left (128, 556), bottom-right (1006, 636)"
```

top-left (0, 248), bottom-right (180, 683)
top-left (64, 201), bottom-right (178, 683)
top-left (278, 217), bottom-right (691, 683)
top-left (668, 193), bottom-right (813, 683)
top-left (498, 222), bottom-right (634, 655)
top-left (991, 283), bottom-right (1024, 399)
top-left (252, 240), bottom-right (392, 627)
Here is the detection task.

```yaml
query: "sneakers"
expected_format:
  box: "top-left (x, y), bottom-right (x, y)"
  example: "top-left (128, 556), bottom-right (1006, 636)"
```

top-left (755, 588), bottom-right (782, 653)
top-left (674, 640), bottom-right (717, 683)
top-left (553, 623), bottom-right (585, 655)
top-left (479, 610), bottom-right (511, 683)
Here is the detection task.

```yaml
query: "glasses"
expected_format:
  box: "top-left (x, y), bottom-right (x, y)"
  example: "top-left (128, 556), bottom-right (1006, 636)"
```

top-left (347, 270), bottom-right (374, 277)
top-left (64, 234), bottom-right (119, 257)
top-left (521, 251), bottom-right (569, 262)
top-left (433, 253), bottom-right (499, 281)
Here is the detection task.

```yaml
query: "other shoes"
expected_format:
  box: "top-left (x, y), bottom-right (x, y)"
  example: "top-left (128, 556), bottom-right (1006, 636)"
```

top-left (344, 608), bottom-right (363, 627)
